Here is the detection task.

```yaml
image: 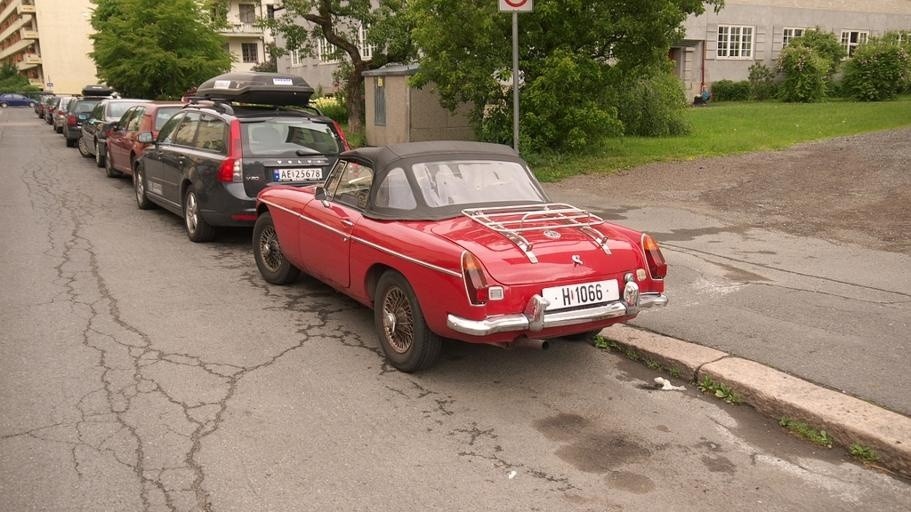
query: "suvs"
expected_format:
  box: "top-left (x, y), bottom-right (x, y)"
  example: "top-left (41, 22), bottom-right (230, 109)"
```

top-left (132, 71), bottom-right (351, 243)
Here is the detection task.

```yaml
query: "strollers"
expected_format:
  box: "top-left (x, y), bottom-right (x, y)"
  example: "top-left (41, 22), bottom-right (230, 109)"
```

top-left (693, 92), bottom-right (710, 104)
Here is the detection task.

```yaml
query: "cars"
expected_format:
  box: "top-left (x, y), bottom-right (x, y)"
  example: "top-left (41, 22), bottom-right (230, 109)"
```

top-left (34, 85), bottom-right (212, 187)
top-left (251, 140), bottom-right (670, 374)
top-left (0, 92), bottom-right (39, 108)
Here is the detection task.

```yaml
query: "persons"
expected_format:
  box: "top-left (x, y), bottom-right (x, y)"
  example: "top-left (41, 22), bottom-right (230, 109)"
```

top-left (701, 81), bottom-right (711, 107)
